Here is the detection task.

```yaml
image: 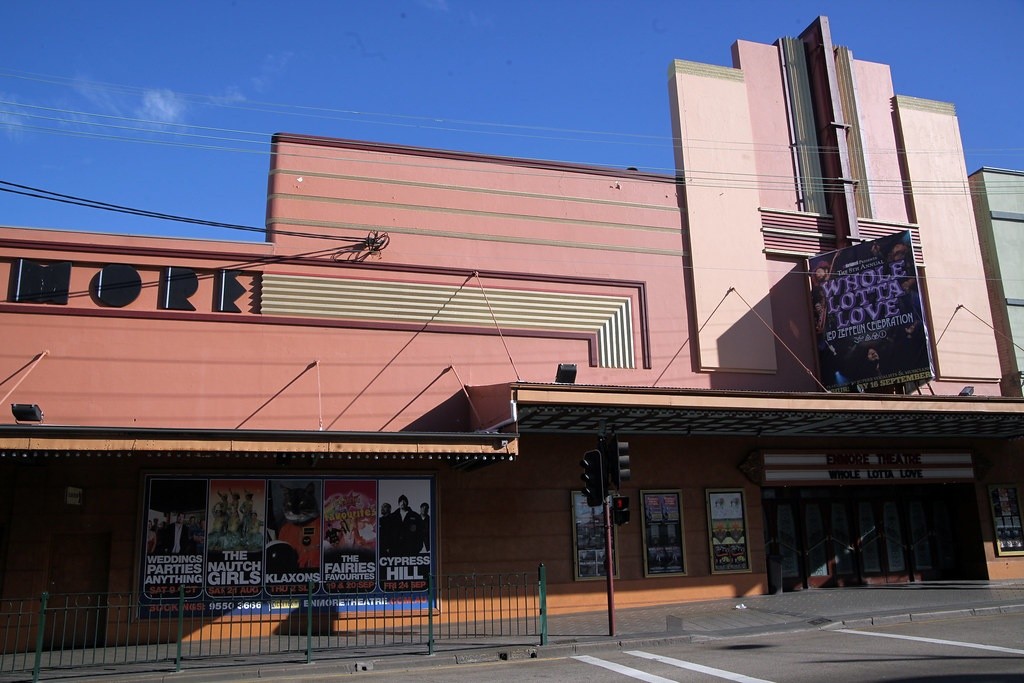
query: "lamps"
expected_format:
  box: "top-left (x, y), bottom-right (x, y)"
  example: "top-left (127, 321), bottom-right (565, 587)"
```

top-left (9, 401), bottom-right (44, 423)
top-left (959, 385), bottom-right (974, 395)
top-left (553, 361), bottom-right (579, 382)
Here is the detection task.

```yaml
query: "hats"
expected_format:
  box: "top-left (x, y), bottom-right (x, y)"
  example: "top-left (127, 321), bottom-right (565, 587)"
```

top-left (398, 495), bottom-right (408, 504)
top-left (420, 503), bottom-right (429, 509)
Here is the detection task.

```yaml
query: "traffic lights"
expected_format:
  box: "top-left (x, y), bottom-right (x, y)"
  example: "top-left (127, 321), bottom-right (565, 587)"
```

top-left (612, 494), bottom-right (631, 526)
top-left (579, 450), bottom-right (603, 507)
top-left (608, 434), bottom-right (631, 491)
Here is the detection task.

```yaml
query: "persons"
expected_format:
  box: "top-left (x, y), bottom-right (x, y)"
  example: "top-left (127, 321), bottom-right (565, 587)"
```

top-left (147, 510), bottom-right (205, 598)
top-left (208, 485), bottom-right (263, 550)
top-left (380, 494), bottom-right (430, 557)
top-left (809, 244), bottom-right (919, 389)
top-left (324, 520), bottom-right (352, 544)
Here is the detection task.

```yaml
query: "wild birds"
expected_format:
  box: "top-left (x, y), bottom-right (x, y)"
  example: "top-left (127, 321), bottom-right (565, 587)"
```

top-left (283, 181), bottom-right (810, 209)
